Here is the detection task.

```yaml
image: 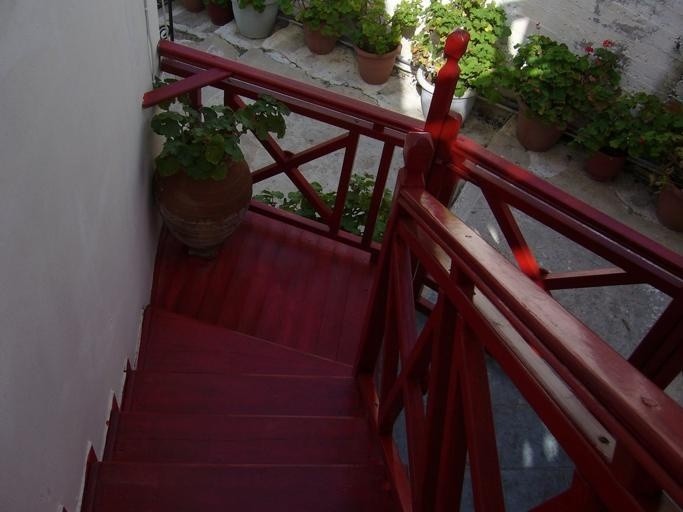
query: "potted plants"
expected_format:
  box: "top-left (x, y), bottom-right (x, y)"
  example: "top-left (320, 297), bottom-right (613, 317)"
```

top-left (145, 74), bottom-right (290, 249)
top-left (295, 2), bottom-right (420, 85)
top-left (569, 90), bottom-right (683, 231)
top-left (410, 1), bottom-right (625, 152)
top-left (231, 2), bottom-right (279, 38)
